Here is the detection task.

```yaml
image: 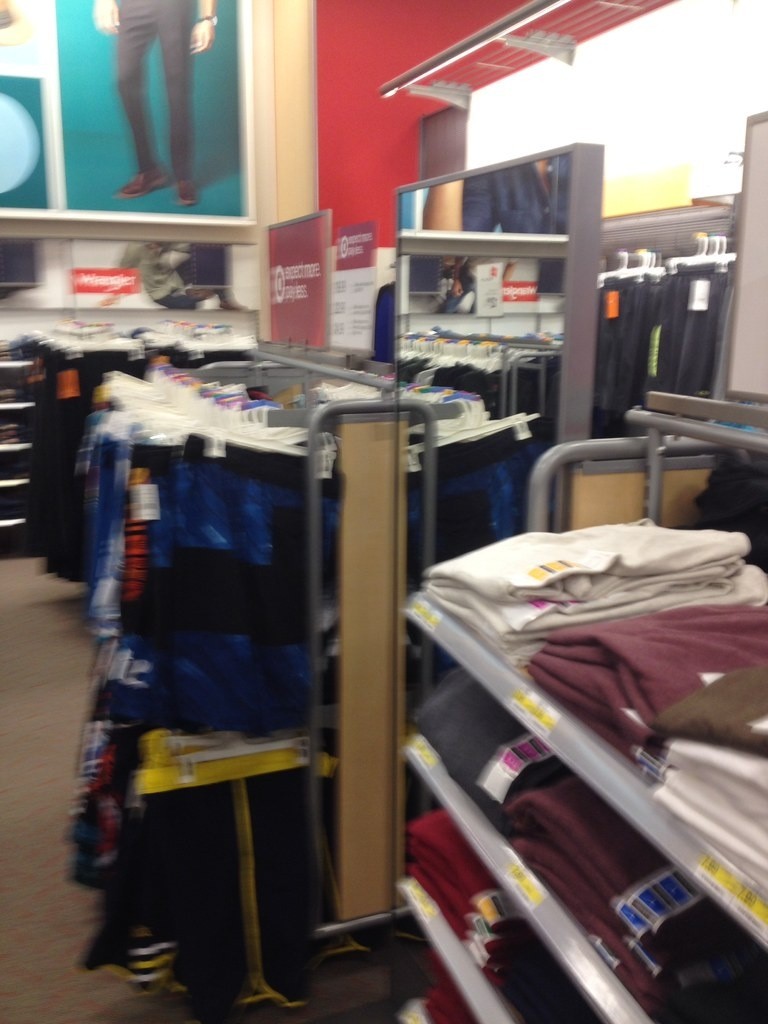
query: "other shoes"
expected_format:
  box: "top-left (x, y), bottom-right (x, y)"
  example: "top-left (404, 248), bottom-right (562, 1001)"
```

top-left (178, 177), bottom-right (198, 204)
top-left (120, 162), bottom-right (168, 196)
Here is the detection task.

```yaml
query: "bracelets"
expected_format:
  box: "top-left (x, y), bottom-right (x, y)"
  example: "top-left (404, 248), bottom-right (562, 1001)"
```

top-left (197, 16), bottom-right (218, 25)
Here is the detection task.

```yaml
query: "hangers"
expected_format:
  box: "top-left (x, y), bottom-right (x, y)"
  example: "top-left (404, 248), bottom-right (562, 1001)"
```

top-left (597, 232), bottom-right (739, 288)
top-left (17, 321), bottom-right (562, 477)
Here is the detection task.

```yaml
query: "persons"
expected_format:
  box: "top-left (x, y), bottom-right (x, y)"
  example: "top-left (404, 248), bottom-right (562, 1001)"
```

top-left (102, 241), bottom-right (246, 311)
top-left (423, 153), bottom-right (571, 236)
top-left (92, 0), bottom-right (219, 203)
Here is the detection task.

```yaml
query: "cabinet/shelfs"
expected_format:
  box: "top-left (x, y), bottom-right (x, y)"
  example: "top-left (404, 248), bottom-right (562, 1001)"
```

top-left (398, 595), bottom-right (768, 1024)
